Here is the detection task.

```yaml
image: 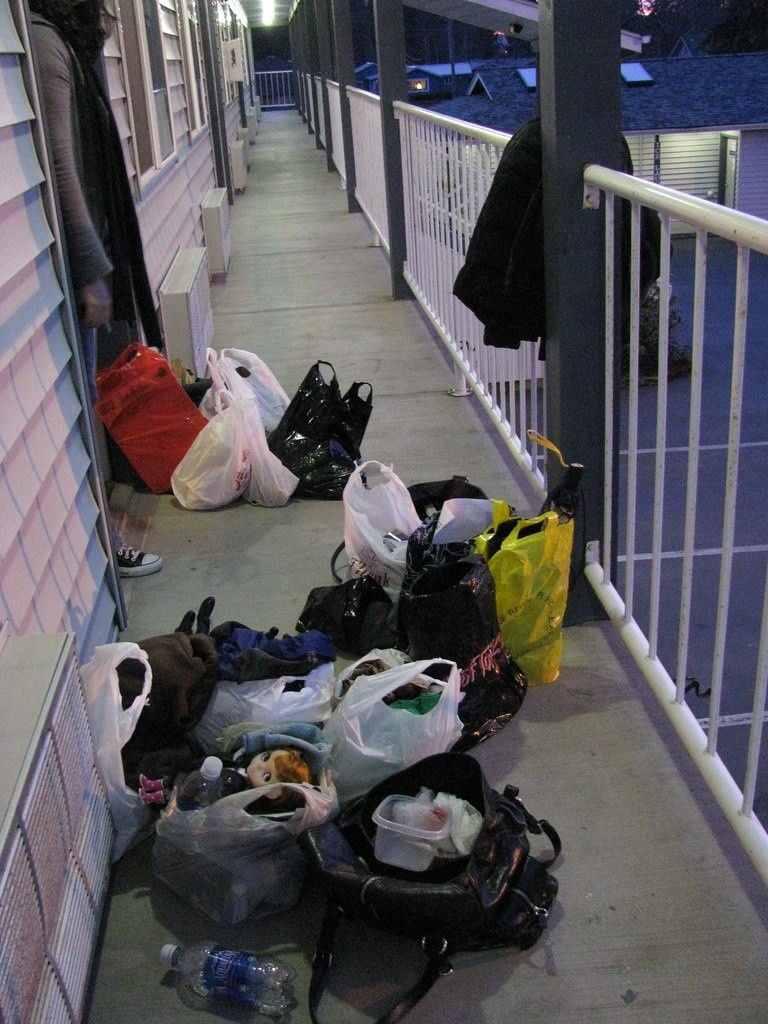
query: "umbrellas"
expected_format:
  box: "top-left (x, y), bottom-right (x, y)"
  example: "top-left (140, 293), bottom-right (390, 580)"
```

top-left (538, 462), bottom-right (585, 531)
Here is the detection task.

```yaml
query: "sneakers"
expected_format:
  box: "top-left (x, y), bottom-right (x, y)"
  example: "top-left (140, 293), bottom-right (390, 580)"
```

top-left (115, 545), bottom-right (162, 577)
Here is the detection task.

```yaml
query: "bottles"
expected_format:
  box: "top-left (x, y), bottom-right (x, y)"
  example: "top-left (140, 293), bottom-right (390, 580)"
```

top-left (165, 757), bottom-right (223, 819)
top-left (161, 941), bottom-right (297, 1016)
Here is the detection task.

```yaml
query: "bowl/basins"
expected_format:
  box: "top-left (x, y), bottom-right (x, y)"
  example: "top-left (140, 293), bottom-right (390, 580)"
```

top-left (372, 794), bottom-right (452, 871)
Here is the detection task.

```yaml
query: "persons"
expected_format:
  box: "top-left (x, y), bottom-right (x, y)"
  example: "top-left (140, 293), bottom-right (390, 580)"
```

top-left (138, 748), bottom-right (309, 821)
top-left (25, 1), bottom-right (162, 578)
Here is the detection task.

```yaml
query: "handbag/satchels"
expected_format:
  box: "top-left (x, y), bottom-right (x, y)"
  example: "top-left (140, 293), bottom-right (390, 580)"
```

top-left (86, 343), bottom-right (582, 1024)
